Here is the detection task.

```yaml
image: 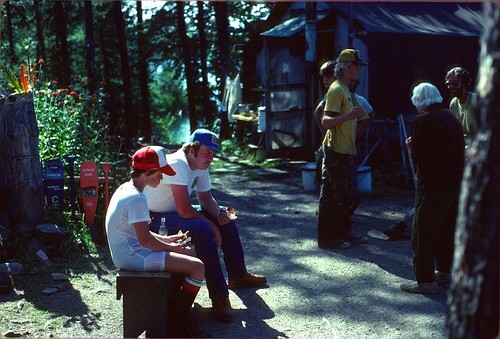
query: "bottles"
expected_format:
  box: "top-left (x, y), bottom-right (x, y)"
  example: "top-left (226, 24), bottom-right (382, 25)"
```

top-left (159, 217), bottom-right (168, 237)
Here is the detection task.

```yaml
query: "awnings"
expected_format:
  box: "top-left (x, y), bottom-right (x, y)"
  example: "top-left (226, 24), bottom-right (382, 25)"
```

top-left (260, 14), bottom-right (336, 37)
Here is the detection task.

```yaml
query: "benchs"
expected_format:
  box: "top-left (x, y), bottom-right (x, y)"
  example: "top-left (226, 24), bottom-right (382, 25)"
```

top-left (116, 271), bottom-right (171, 339)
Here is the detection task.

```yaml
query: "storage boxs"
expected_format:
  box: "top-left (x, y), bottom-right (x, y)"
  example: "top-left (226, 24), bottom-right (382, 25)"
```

top-left (301, 161), bottom-right (373, 195)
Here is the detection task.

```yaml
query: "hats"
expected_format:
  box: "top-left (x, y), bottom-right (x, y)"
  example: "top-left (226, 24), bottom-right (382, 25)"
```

top-left (338, 48), bottom-right (366, 66)
top-left (132, 146), bottom-right (176, 176)
top-left (189, 128), bottom-right (222, 152)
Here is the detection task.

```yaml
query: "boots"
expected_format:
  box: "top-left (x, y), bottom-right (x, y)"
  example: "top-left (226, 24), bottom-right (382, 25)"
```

top-left (167, 285), bottom-right (209, 338)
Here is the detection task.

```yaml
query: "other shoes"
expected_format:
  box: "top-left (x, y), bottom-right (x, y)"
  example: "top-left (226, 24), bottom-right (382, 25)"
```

top-left (319, 238), bottom-right (351, 248)
top-left (434, 273), bottom-right (450, 287)
top-left (401, 282), bottom-right (437, 293)
top-left (229, 273), bottom-right (267, 286)
top-left (213, 297), bottom-right (236, 322)
top-left (344, 232), bottom-right (367, 243)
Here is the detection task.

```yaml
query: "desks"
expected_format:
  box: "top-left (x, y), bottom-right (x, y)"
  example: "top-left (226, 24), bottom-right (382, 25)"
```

top-left (232, 114), bottom-right (266, 161)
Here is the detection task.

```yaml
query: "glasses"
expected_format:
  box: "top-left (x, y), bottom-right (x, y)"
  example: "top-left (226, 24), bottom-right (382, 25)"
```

top-left (445, 80), bottom-right (464, 86)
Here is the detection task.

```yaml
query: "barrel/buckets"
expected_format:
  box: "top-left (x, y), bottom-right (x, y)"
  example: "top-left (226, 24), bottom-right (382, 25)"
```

top-left (356, 165), bottom-right (372, 193)
top-left (257, 106), bottom-right (266, 132)
top-left (301, 165), bottom-right (318, 191)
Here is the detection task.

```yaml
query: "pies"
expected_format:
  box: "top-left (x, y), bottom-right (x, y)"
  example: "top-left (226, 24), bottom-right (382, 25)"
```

top-left (219, 207), bottom-right (236, 220)
top-left (177, 229), bottom-right (189, 241)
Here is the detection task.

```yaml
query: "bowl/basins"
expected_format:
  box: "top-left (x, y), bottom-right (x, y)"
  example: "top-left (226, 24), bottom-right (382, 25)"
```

top-left (238, 103), bottom-right (255, 114)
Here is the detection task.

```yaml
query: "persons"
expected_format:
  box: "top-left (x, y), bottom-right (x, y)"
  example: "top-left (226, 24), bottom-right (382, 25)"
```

top-left (105, 146), bottom-right (211, 339)
top-left (314, 49), bottom-right (376, 248)
top-left (445, 67), bottom-right (478, 150)
top-left (402, 82), bottom-right (465, 293)
top-left (143, 129), bottom-right (267, 320)
